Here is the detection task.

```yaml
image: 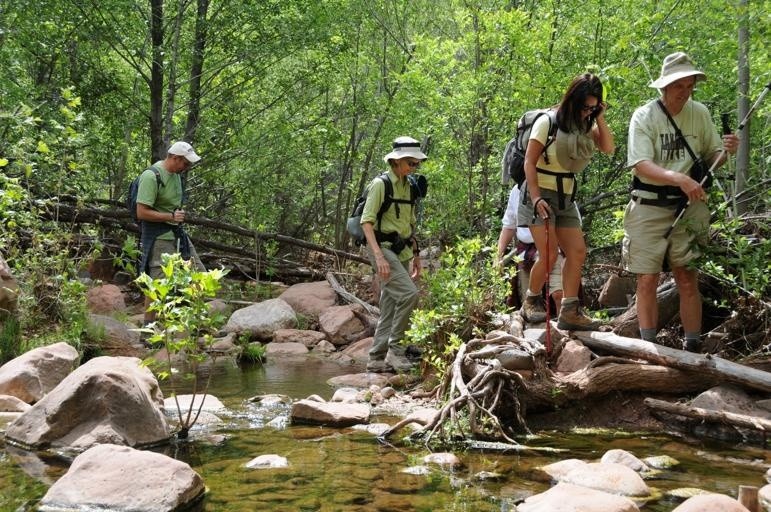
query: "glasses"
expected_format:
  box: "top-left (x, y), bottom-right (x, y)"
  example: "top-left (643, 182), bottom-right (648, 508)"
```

top-left (582, 104), bottom-right (597, 111)
top-left (404, 162), bottom-right (420, 167)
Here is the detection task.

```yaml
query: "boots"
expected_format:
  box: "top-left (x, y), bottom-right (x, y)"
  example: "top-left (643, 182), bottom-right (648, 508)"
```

top-left (683, 339), bottom-right (702, 353)
top-left (557, 302), bottom-right (603, 331)
top-left (519, 293), bottom-right (547, 322)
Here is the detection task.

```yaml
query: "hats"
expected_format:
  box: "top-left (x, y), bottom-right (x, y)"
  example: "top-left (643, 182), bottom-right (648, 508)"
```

top-left (647, 51), bottom-right (707, 88)
top-left (557, 121), bottom-right (596, 171)
top-left (169, 141), bottom-right (201, 163)
top-left (383, 136), bottom-right (428, 162)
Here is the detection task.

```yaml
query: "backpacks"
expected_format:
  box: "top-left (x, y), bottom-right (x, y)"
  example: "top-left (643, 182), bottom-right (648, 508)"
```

top-left (128, 167), bottom-right (161, 221)
top-left (347, 175), bottom-right (414, 245)
top-left (508, 109), bottom-right (558, 188)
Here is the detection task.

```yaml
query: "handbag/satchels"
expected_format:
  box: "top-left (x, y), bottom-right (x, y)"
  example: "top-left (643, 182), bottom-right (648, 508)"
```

top-left (692, 161), bottom-right (712, 188)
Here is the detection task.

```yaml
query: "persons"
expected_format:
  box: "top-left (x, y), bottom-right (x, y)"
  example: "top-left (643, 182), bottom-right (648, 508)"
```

top-left (134, 140), bottom-right (214, 350)
top-left (622, 50), bottom-right (741, 352)
top-left (517, 72), bottom-right (616, 332)
top-left (492, 180), bottom-right (582, 316)
top-left (361, 135), bottom-right (432, 373)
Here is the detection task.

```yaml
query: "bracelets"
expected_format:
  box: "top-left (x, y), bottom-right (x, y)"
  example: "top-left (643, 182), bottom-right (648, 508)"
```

top-left (413, 249), bottom-right (421, 255)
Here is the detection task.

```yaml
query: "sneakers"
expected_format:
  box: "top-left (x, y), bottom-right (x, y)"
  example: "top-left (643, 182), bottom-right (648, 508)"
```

top-left (366, 351), bottom-right (413, 372)
top-left (139, 332), bottom-right (165, 349)
